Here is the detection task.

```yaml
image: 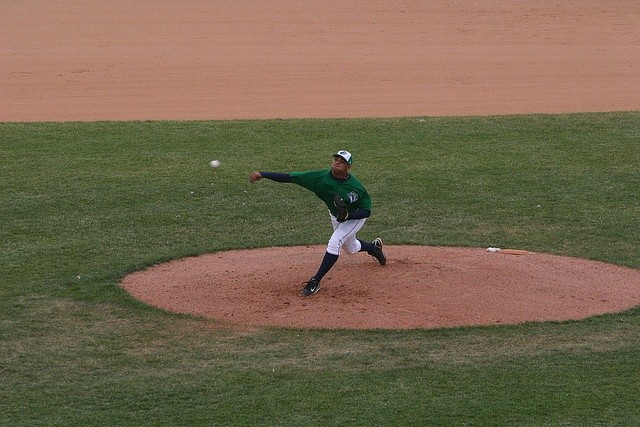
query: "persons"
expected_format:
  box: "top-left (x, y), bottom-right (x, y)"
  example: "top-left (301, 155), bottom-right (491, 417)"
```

top-left (249, 150), bottom-right (386, 296)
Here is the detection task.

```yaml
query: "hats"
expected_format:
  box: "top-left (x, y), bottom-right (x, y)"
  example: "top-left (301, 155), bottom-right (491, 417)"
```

top-left (332, 150), bottom-right (353, 165)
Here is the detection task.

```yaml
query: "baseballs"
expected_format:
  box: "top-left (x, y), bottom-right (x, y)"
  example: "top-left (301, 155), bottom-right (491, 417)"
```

top-left (208, 160), bottom-right (220, 170)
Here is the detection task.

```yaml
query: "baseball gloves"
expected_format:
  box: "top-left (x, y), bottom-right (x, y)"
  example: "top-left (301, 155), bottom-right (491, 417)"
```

top-left (332, 194), bottom-right (348, 223)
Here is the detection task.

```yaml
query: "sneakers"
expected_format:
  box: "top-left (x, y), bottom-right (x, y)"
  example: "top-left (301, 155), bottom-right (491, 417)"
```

top-left (302, 277), bottom-right (321, 296)
top-left (370, 238), bottom-right (386, 265)
